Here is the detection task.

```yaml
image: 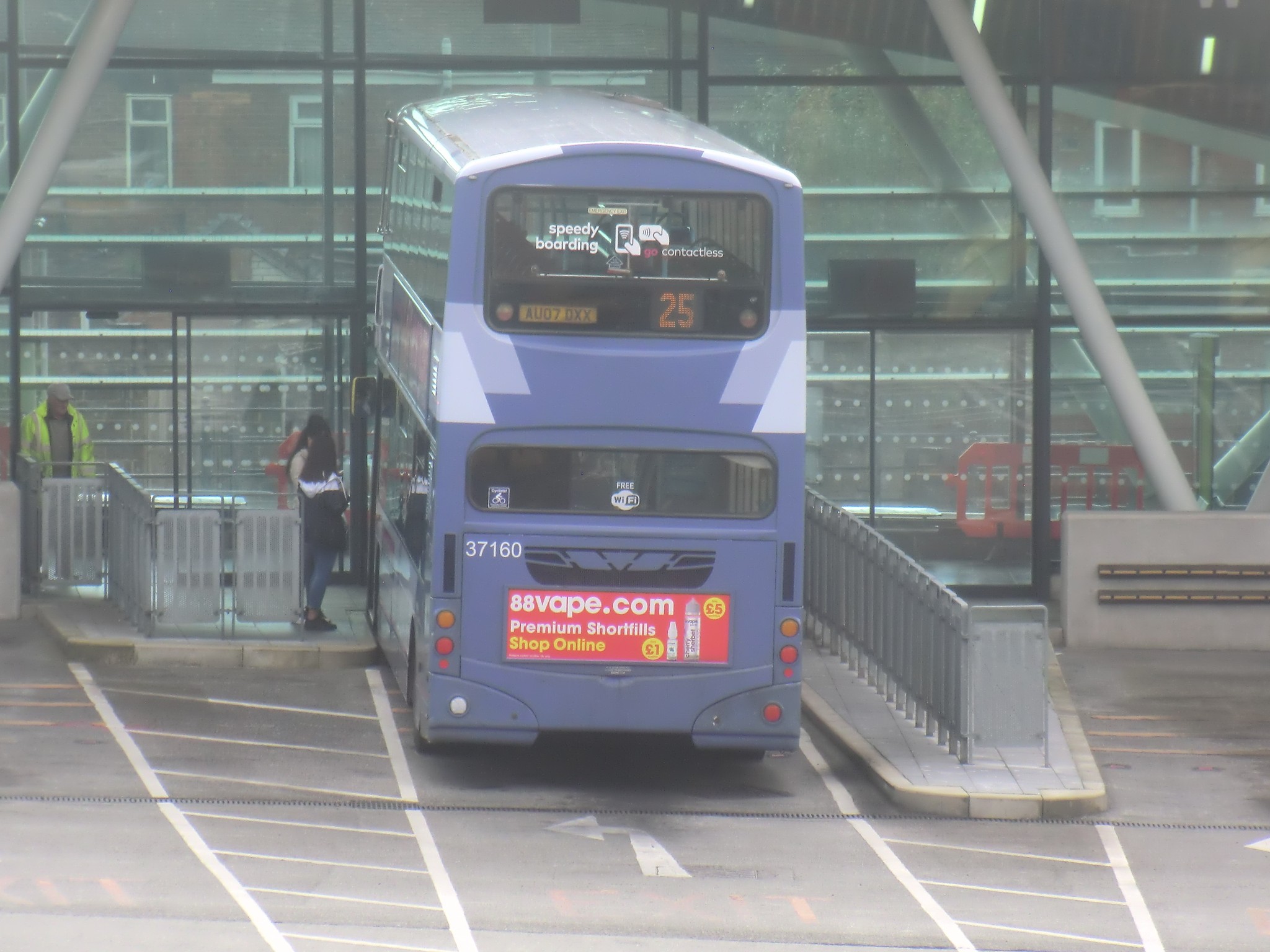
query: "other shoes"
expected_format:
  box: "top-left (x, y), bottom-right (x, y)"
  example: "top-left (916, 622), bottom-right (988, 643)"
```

top-left (299, 606), bottom-right (327, 620)
top-left (304, 614), bottom-right (337, 631)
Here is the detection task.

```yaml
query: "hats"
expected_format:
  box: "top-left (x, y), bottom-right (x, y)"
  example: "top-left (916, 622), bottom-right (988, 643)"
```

top-left (48, 383), bottom-right (74, 401)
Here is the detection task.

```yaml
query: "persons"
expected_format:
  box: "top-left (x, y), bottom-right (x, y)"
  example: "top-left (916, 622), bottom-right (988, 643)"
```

top-left (21, 382), bottom-right (96, 479)
top-left (286, 414), bottom-right (351, 632)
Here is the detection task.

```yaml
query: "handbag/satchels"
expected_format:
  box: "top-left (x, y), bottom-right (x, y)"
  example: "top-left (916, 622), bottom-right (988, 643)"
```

top-left (338, 513), bottom-right (350, 550)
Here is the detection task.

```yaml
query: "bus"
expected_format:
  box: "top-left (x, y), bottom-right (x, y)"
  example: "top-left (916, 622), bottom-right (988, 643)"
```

top-left (346, 86), bottom-right (811, 770)
